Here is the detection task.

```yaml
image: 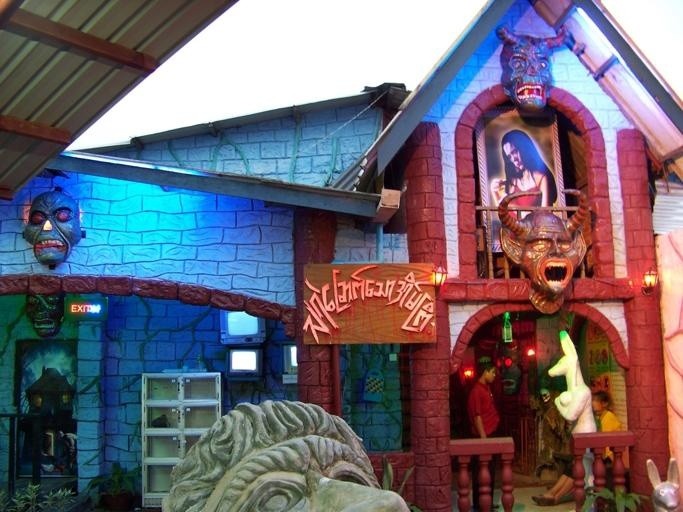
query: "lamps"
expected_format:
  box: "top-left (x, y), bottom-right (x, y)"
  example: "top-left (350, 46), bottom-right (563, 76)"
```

top-left (641, 263), bottom-right (658, 295)
top-left (433, 260), bottom-right (447, 292)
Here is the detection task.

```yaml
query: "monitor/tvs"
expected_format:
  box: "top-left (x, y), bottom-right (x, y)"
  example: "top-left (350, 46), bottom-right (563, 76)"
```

top-left (283, 346), bottom-right (298, 373)
top-left (226, 349), bottom-right (260, 381)
top-left (220, 309), bottom-right (266, 343)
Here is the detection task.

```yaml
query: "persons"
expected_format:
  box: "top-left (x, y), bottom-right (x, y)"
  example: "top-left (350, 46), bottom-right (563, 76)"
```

top-left (490, 128), bottom-right (558, 224)
top-left (529, 389), bottom-right (622, 506)
top-left (468, 360), bottom-right (501, 511)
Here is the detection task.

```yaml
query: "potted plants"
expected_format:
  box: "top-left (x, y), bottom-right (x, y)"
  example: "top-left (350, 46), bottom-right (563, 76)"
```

top-left (88, 460), bottom-right (140, 512)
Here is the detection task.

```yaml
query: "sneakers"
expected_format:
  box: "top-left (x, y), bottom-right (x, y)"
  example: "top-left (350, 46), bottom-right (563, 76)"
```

top-left (532, 494), bottom-right (557, 506)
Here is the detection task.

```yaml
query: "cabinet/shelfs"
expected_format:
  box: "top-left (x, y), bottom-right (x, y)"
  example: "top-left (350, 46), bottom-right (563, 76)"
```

top-left (141, 371), bottom-right (221, 508)
